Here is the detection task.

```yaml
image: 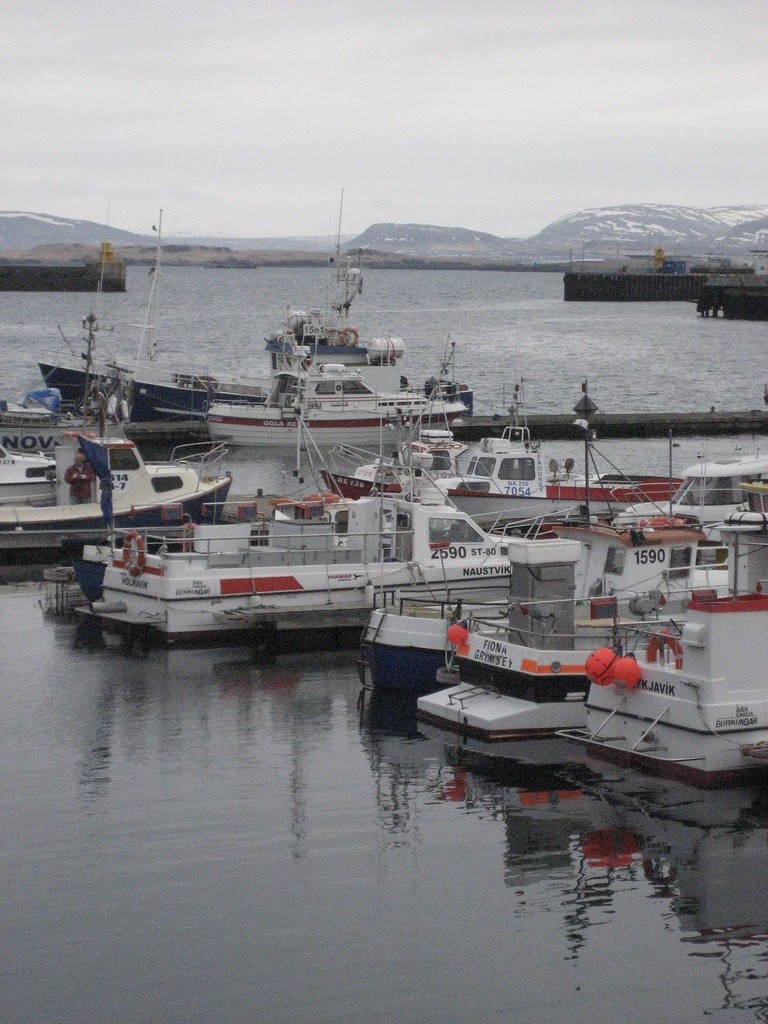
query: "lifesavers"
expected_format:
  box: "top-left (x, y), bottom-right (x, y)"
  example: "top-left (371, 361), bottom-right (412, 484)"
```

top-left (177, 522), bottom-right (199, 547)
top-left (340, 327), bottom-right (359, 346)
top-left (302, 493), bottom-right (342, 504)
top-left (79, 390), bottom-right (109, 421)
top-left (640, 516), bottom-right (685, 529)
top-left (646, 629), bottom-right (684, 670)
top-left (121, 531), bottom-right (144, 575)
top-left (64, 431), bottom-right (95, 439)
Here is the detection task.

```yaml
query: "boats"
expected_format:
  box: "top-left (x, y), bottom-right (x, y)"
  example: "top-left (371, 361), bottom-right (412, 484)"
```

top-left (0, 187), bottom-right (767, 767)
top-left (561, 476), bottom-right (768, 790)
top-left (697, 248), bottom-right (768, 319)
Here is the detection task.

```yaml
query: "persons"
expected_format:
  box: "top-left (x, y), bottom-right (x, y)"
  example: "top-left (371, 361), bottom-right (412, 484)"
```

top-left (64, 453), bottom-right (96, 505)
top-left (178, 515), bottom-right (198, 553)
